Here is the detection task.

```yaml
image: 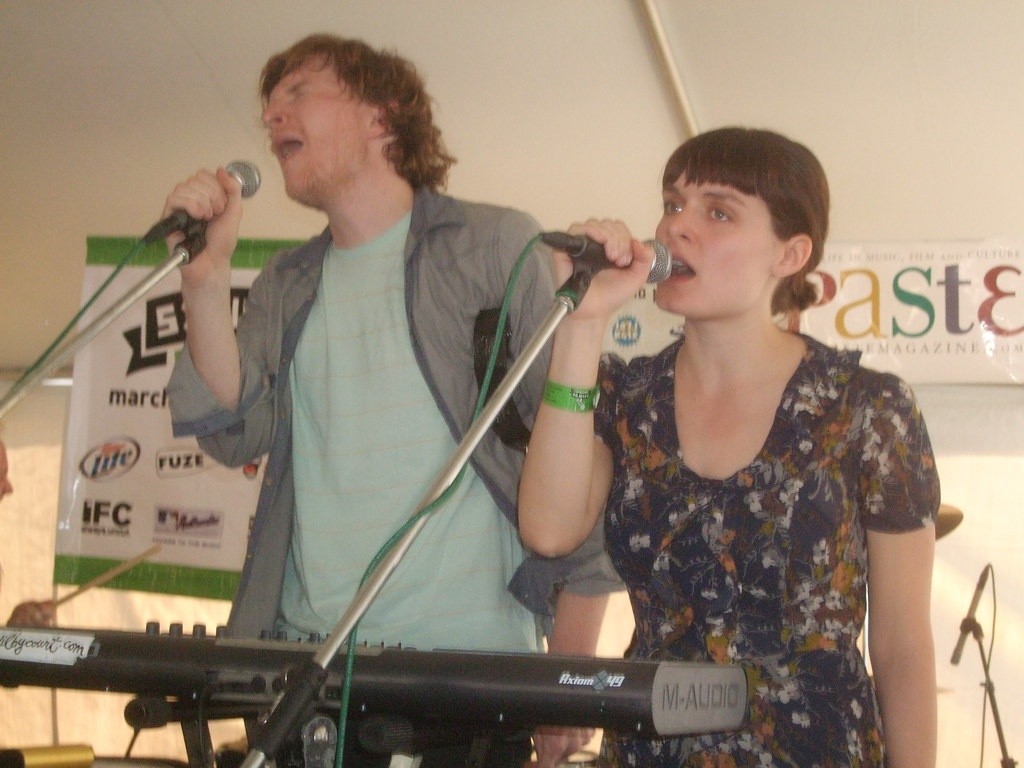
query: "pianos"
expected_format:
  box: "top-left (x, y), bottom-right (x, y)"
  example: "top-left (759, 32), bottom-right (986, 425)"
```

top-left (2, 620), bottom-right (751, 741)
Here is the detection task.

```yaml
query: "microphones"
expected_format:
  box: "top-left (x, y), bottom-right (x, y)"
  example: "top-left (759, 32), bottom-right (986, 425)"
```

top-left (539, 232), bottom-right (672, 283)
top-left (141, 159), bottom-right (262, 241)
top-left (951, 567), bottom-right (989, 665)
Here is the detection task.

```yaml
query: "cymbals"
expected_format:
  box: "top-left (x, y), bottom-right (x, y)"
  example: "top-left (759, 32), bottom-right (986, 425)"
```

top-left (932, 502), bottom-right (962, 542)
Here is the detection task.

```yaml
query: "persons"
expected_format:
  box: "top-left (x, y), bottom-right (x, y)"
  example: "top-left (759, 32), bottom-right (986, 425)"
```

top-left (515, 127), bottom-right (936, 768)
top-left (164, 36), bottom-right (617, 767)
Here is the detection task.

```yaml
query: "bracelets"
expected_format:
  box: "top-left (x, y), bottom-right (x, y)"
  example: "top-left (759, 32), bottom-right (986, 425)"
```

top-left (540, 379), bottom-right (598, 412)
